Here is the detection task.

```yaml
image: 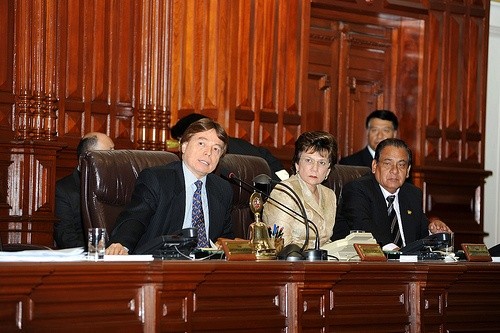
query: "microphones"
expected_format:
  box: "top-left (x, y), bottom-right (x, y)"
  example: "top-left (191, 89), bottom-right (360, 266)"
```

top-left (254, 174), bottom-right (310, 260)
top-left (220, 169), bottom-right (327, 261)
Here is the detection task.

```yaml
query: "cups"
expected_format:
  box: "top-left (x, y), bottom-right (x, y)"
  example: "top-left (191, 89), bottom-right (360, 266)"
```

top-left (88, 227), bottom-right (106, 260)
top-left (267, 238), bottom-right (284, 255)
top-left (440, 231), bottom-right (454, 258)
top-left (350, 230), bottom-right (365, 233)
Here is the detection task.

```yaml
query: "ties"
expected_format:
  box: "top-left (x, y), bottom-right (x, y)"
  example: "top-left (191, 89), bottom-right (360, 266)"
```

top-left (191, 180), bottom-right (210, 249)
top-left (386, 195), bottom-right (403, 249)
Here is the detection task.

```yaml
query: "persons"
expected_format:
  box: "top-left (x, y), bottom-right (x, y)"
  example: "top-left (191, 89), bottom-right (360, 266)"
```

top-left (54, 132), bottom-right (115, 250)
top-left (105, 119), bottom-right (237, 259)
top-left (329, 138), bottom-right (454, 252)
top-left (339, 110), bottom-right (399, 167)
top-left (171, 113), bottom-right (284, 189)
top-left (262, 130), bottom-right (336, 250)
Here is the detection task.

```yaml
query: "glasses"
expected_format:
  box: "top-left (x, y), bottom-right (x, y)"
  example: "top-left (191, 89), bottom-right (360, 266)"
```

top-left (377, 161), bottom-right (408, 170)
